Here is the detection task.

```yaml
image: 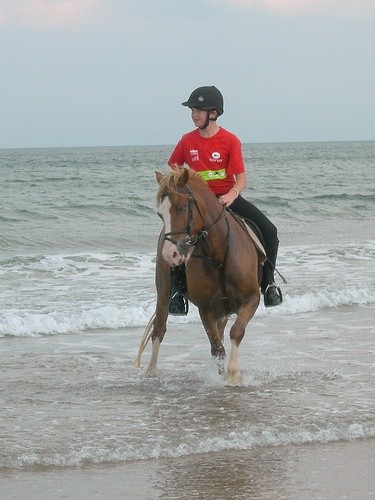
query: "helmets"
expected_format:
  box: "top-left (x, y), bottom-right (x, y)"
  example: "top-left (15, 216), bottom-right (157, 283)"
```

top-left (181, 85), bottom-right (223, 115)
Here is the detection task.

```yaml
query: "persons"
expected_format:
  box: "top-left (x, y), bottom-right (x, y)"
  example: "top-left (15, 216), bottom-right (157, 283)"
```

top-left (168, 86), bottom-right (282, 315)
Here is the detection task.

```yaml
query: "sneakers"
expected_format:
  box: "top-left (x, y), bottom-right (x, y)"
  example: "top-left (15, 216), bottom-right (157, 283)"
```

top-left (168, 292), bottom-right (184, 313)
top-left (260, 283), bottom-right (280, 305)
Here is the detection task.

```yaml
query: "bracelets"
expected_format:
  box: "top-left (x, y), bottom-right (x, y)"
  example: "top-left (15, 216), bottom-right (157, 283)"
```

top-left (229, 188), bottom-right (239, 199)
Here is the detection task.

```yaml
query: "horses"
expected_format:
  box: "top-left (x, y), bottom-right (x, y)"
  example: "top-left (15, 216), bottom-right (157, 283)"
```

top-left (136, 167), bottom-right (265, 386)
